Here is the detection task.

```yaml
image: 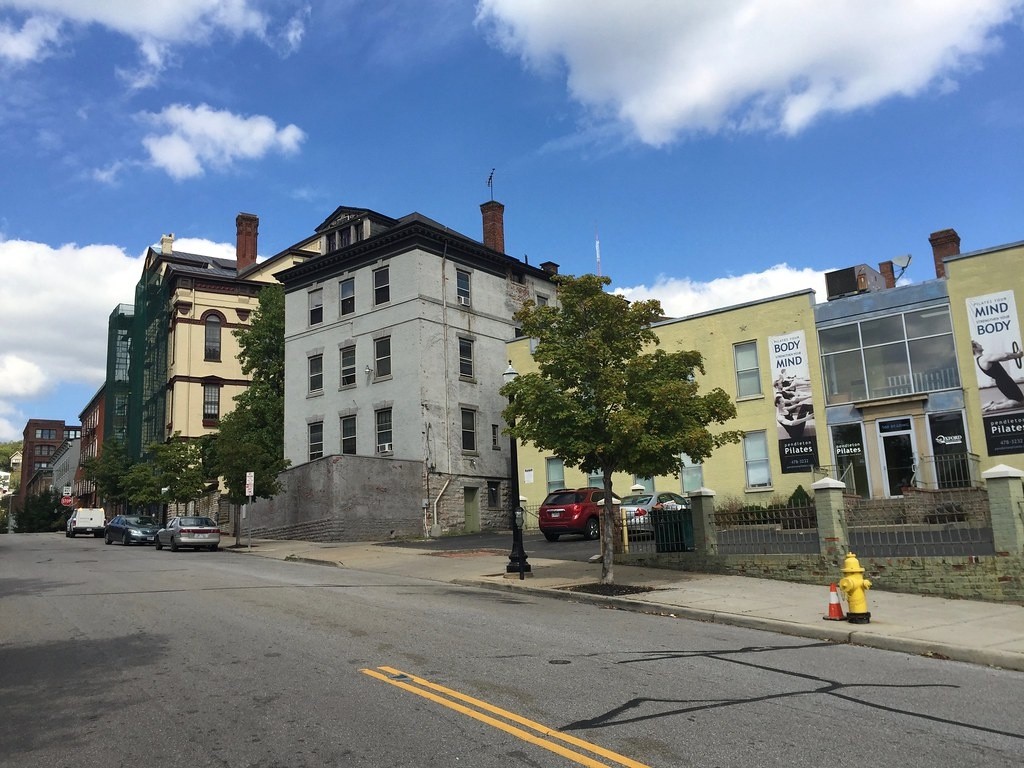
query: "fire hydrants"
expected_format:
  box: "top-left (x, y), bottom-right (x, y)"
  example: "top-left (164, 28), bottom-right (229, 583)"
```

top-left (838, 552), bottom-right (872, 624)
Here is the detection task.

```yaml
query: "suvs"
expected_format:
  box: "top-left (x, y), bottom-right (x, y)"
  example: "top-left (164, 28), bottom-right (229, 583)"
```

top-left (538, 486), bottom-right (623, 542)
top-left (66, 507), bottom-right (107, 539)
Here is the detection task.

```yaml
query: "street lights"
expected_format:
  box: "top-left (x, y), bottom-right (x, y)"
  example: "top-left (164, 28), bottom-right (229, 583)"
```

top-left (501, 359), bottom-right (536, 580)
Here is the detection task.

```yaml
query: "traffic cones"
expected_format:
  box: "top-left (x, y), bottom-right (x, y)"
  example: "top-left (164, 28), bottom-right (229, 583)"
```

top-left (822, 583), bottom-right (847, 621)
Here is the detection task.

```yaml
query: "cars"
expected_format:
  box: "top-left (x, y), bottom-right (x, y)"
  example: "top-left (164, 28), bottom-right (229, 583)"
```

top-left (104, 515), bottom-right (163, 547)
top-left (154, 516), bottom-right (221, 554)
top-left (618, 491), bottom-right (692, 539)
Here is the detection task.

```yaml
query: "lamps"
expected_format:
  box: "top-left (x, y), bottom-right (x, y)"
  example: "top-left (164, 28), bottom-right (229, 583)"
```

top-left (365, 365), bottom-right (373, 374)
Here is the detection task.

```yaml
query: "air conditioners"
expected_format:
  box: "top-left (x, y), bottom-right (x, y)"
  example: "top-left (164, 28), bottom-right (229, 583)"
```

top-left (459, 297), bottom-right (470, 307)
top-left (379, 444), bottom-right (391, 452)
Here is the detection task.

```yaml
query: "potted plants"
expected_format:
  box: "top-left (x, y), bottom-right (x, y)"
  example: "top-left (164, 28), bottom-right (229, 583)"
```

top-left (895, 479), bottom-right (909, 492)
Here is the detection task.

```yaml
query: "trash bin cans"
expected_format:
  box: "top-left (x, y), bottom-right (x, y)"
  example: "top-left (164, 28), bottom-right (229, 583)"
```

top-left (647, 505), bottom-right (695, 553)
